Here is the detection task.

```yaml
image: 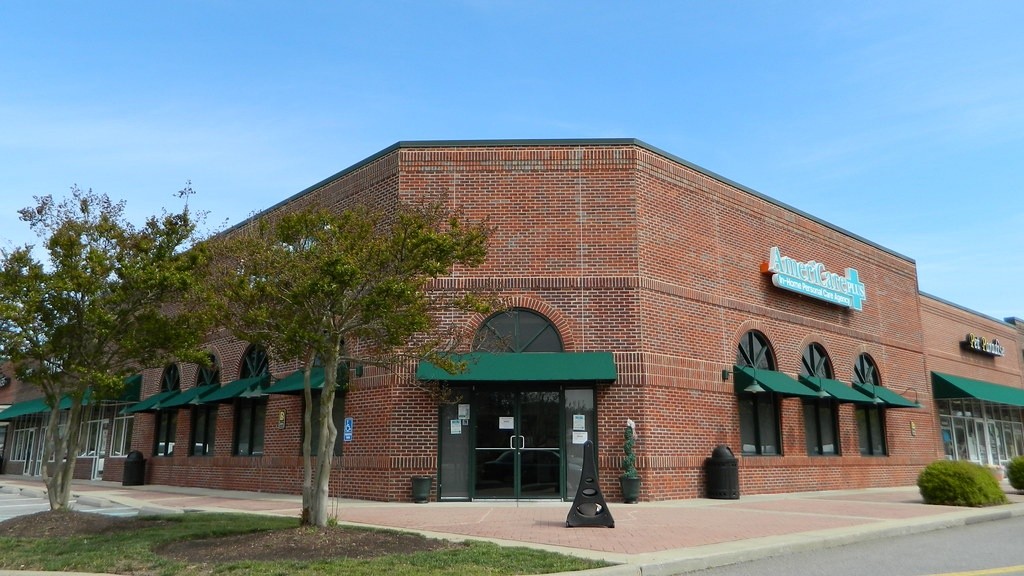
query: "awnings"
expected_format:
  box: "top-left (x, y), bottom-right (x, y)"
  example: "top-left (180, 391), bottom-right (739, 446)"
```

top-left (853, 383), bottom-right (923, 407)
top-left (196, 374), bottom-right (270, 403)
top-left (262, 366), bottom-right (346, 394)
top-left (0, 390), bottom-right (97, 420)
top-left (798, 373), bottom-right (875, 402)
top-left (159, 384), bottom-right (221, 408)
top-left (416, 351), bottom-right (617, 381)
top-left (734, 366), bottom-right (816, 396)
top-left (931, 370), bottom-right (1024, 406)
top-left (123, 390), bottom-right (180, 413)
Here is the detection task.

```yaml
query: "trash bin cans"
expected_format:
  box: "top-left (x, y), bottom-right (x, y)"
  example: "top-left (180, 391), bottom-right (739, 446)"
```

top-left (706, 445), bottom-right (740, 500)
top-left (122, 451), bottom-right (145, 486)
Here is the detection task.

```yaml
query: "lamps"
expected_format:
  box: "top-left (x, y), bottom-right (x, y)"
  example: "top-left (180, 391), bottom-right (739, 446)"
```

top-left (150, 388), bottom-right (178, 411)
top-left (800, 373), bottom-right (831, 398)
top-left (118, 396), bottom-right (142, 415)
top-left (856, 378), bottom-right (884, 405)
top-left (723, 362), bottom-right (765, 395)
top-left (898, 386), bottom-right (926, 408)
top-left (188, 381), bottom-right (215, 407)
top-left (355, 364), bottom-right (363, 377)
top-left (240, 372), bottom-right (258, 400)
top-left (252, 371), bottom-right (280, 398)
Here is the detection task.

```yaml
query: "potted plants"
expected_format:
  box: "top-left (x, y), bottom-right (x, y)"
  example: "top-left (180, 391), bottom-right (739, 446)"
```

top-left (619, 426), bottom-right (642, 504)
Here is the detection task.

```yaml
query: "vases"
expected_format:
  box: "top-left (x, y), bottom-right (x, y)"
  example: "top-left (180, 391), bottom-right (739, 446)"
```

top-left (410, 476), bottom-right (435, 504)
